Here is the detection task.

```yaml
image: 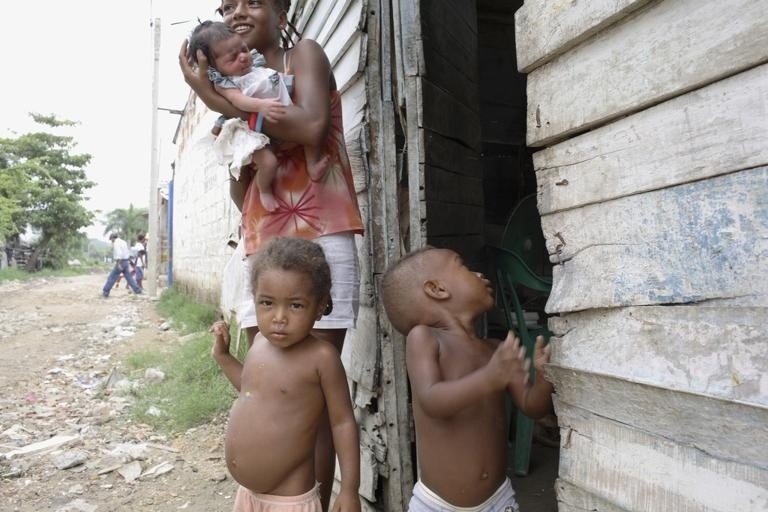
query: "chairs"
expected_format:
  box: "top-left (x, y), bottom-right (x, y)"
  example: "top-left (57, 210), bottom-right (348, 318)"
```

top-left (489, 242), bottom-right (552, 478)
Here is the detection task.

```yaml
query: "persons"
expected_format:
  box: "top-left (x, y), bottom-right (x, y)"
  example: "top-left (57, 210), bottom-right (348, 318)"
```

top-left (186, 21), bottom-right (330, 211)
top-left (381, 246), bottom-right (555, 512)
top-left (103, 233), bottom-right (146, 297)
top-left (178, 1), bottom-right (366, 512)
top-left (209, 237), bottom-right (360, 512)
